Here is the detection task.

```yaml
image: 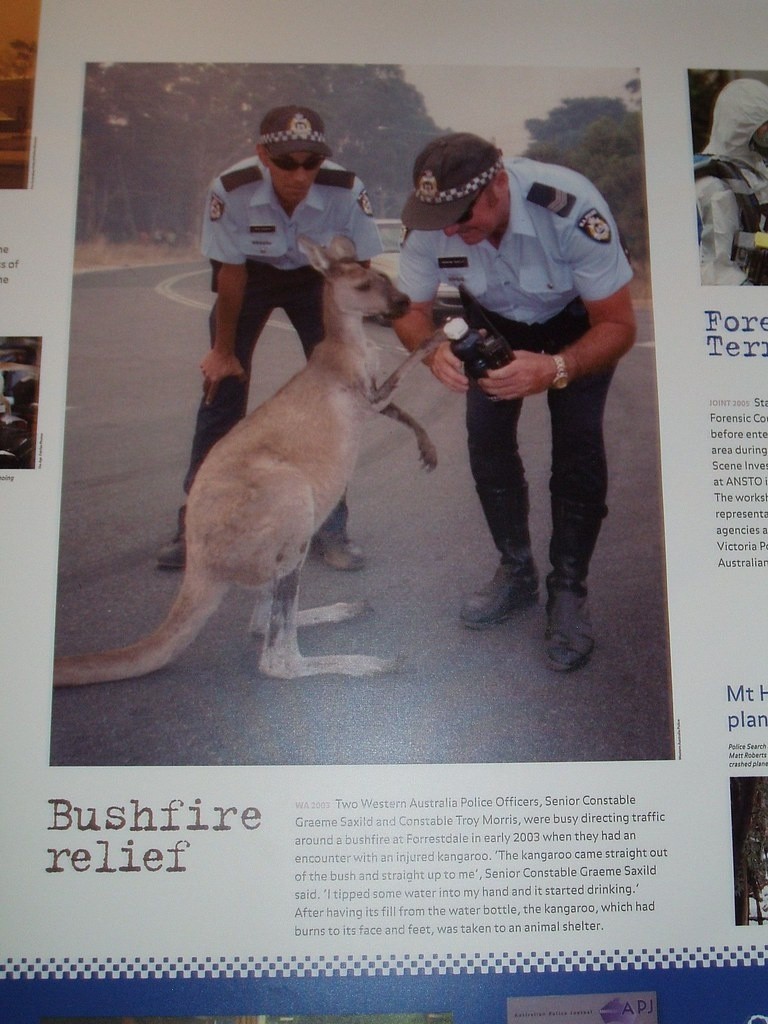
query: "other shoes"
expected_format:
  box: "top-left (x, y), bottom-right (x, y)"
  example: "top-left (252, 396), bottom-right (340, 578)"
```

top-left (158, 533), bottom-right (187, 568)
top-left (318, 526), bottom-right (366, 568)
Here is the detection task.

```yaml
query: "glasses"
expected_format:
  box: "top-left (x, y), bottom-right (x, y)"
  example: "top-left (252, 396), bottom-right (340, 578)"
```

top-left (456, 185), bottom-right (487, 223)
top-left (270, 153), bottom-right (321, 171)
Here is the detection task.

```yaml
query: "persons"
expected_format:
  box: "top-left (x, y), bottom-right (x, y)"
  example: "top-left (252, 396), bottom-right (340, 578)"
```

top-left (693, 78), bottom-right (768, 287)
top-left (156, 105), bottom-right (385, 567)
top-left (394, 131), bottom-right (637, 673)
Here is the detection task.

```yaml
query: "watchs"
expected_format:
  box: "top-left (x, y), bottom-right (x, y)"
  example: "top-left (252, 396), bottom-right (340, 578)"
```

top-left (552, 355), bottom-right (568, 388)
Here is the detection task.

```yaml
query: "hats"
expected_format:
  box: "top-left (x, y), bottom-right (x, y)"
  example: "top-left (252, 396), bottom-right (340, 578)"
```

top-left (399, 133), bottom-right (503, 232)
top-left (259, 105), bottom-right (333, 157)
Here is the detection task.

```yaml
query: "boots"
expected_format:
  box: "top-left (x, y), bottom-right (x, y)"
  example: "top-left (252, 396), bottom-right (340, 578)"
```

top-left (543, 496), bottom-right (609, 670)
top-left (459, 482), bottom-right (540, 626)
